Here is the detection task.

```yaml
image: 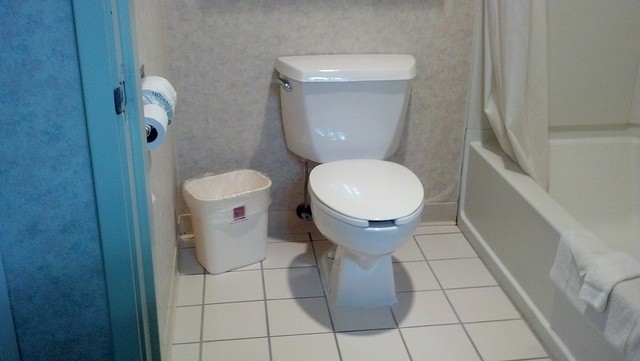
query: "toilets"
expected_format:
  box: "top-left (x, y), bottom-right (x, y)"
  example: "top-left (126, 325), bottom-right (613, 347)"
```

top-left (275, 52), bottom-right (426, 310)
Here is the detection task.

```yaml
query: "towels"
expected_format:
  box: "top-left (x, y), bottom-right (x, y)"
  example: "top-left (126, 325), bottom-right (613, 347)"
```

top-left (602, 276), bottom-right (640, 360)
top-left (548, 229), bottom-right (608, 318)
top-left (578, 251), bottom-right (640, 312)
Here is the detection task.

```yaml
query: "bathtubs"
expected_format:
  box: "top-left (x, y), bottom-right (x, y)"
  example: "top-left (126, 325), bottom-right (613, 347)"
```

top-left (457, 130), bottom-right (640, 361)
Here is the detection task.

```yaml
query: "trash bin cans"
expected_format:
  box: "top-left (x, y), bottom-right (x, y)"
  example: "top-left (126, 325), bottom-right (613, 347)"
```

top-left (182, 167), bottom-right (274, 276)
